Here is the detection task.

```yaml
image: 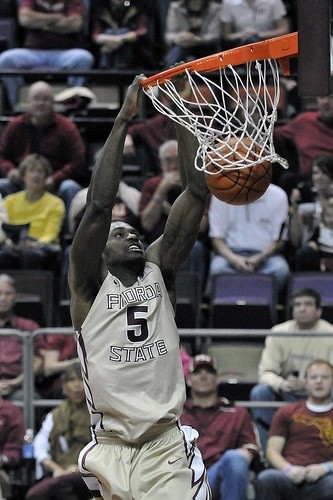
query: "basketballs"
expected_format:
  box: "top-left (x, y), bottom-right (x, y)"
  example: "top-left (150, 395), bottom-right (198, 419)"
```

top-left (201, 137), bottom-right (272, 205)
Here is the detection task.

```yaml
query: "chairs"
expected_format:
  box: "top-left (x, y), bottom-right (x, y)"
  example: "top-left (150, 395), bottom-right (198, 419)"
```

top-left (0, 20), bottom-right (333, 384)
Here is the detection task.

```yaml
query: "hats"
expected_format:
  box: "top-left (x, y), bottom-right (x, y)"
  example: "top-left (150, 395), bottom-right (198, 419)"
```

top-left (188, 354), bottom-right (218, 373)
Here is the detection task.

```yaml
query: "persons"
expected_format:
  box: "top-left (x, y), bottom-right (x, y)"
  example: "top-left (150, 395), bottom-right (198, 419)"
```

top-left (0, 0), bottom-right (333, 285)
top-left (0, 272), bottom-right (333, 500)
top-left (69, 71), bottom-right (218, 500)
